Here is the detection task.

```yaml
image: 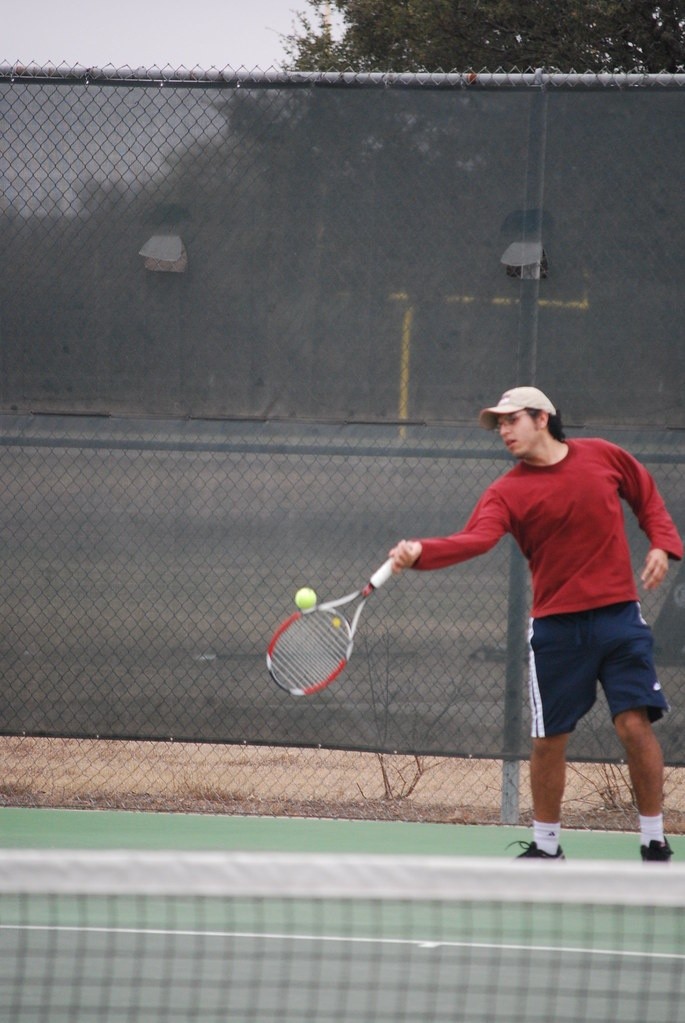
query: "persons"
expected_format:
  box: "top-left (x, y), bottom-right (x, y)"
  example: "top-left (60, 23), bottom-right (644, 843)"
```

top-left (389, 386), bottom-right (685, 863)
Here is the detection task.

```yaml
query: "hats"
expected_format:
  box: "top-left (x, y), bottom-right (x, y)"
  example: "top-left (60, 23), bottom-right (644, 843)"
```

top-left (479, 387), bottom-right (556, 430)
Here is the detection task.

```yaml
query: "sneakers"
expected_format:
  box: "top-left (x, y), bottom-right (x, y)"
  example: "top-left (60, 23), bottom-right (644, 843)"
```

top-left (503, 840), bottom-right (568, 865)
top-left (639, 835), bottom-right (674, 864)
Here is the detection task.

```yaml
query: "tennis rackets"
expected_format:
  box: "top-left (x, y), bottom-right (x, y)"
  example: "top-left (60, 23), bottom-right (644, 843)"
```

top-left (264, 557), bottom-right (396, 698)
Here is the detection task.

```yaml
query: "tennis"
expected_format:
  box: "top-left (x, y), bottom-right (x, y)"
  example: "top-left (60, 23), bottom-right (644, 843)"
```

top-left (294, 587), bottom-right (317, 609)
